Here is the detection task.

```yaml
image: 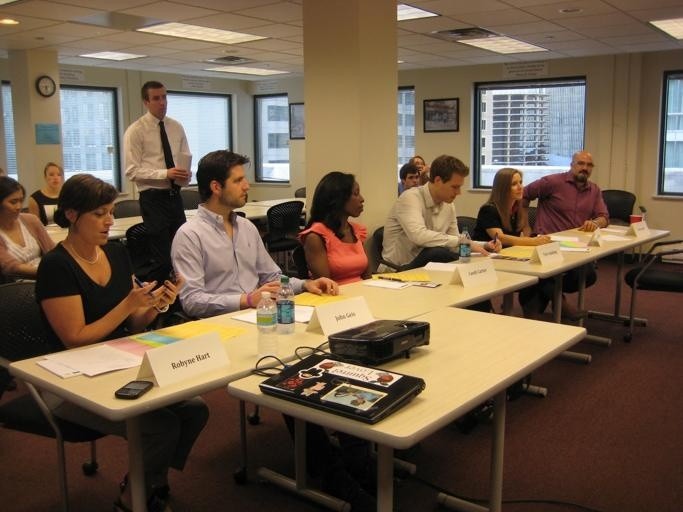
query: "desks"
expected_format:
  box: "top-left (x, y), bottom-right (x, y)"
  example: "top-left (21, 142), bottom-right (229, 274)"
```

top-left (109, 204), bottom-right (267, 241)
top-left (7, 256), bottom-right (548, 511)
top-left (45, 196), bottom-right (128, 285)
top-left (247, 197), bottom-right (307, 214)
top-left (456, 221), bottom-right (671, 364)
top-left (228, 306), bottom-right (588, 511)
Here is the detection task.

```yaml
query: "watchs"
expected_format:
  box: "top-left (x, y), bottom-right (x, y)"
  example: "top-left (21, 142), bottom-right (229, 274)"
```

top-left (155, 305), bottom-right (170, 313)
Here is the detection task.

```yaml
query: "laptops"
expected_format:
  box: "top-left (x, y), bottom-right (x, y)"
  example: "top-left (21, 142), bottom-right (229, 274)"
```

top-left (260, 354), bottom-right (426, 424)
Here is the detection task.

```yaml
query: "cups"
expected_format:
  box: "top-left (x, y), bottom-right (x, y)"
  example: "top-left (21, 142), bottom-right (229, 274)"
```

top-left (630, 214), bottom-right (642, 223)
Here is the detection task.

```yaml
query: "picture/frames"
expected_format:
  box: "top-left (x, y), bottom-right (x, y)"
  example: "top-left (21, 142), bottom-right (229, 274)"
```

top-left (423, 97), bottom-right (459, 133)
top-left (289, 102), bottom-right (306, 140)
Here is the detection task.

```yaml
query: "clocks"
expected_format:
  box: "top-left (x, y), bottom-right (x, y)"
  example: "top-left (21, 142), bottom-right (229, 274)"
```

top-left (36, 75), bottom-right (57, 98)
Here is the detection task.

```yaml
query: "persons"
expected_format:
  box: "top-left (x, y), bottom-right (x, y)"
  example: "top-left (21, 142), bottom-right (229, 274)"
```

top-left (471, 168), bottom-right (555, 322)
top-left (27, 162), bottom-right (69, 227)
top-left (376, 156), bottom-right (502, 312)
top-left (296, 172), bottom-right (368, 285)
top-left (396, 164), bottom-right (421, 193)
top-left (0, 174), bottom-right (58, 281)
top-left (186, 151), bottom-right (402, 498)
top-left (123, 80), bottom-right (192, 262)
top-left (409, 155), bottom-right (426, 177)
top-left (515, 151), bottom-right (609, 319)
top-left (35, 173), bottom-right (210, 512)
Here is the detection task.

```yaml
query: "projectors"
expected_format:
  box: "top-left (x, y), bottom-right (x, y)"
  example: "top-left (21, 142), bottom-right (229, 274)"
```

top-left (328, 319), bottom-right (430, 365)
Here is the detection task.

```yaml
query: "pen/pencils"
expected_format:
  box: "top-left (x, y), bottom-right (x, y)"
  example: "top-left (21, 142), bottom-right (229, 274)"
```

top-left (135, 278), bottom-right (154, 298)
top-left (378, 276), bottom-right (402, 282)
top-left (493, 231), bottom-right (498, 245)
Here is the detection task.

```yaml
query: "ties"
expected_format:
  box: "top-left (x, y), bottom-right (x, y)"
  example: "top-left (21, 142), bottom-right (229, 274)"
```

top-left (159, 121), bottom-right (179, 195)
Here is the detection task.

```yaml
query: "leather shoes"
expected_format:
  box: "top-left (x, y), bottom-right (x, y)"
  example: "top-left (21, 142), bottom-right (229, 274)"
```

top-left (551, 298), bottom-right (587, 322)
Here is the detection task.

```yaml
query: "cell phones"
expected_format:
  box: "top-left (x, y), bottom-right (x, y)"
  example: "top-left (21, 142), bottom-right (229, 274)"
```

top-left (115, 380), bottom-right (153, 399)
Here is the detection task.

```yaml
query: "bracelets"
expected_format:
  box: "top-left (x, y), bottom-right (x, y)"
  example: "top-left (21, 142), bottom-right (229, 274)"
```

top-left (459, 231), bottom-right (469, 240)
top-left (246, 291), bottom-right (257, 309)
top-left (590, 220), bottom-right (600, 227)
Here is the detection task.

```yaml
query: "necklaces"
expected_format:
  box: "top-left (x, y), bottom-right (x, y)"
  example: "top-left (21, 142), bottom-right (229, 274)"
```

top-left (66, 238), bottom-right (99, 263)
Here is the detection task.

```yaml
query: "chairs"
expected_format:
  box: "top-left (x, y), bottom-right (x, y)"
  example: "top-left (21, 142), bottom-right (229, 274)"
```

top-left (361, 226), bottom-right (384, 277)
top-left (113, 199), bottom-right (141, 219)
top-left (0, 281), bottom-right (107, 511)
top-left (456, 215), bottom-right (477, 239)
top-left (146, 263), bottom-right (204, 328)
top-left (180, 190), bottom-right (202, 210)
top-left (512, 206), bottom-right (537, 232)
top-left (294, 187), bottom-right (305, 197)
top-left (120, 222), bottom-right (159, 280)
top-left (257, 200), bottom-right (304, 277)
top-left (601, 190), bottom-right (635, 227)
top-left (624, 240), bottom-right (683, 344)
top-left (292, 245), bottom-right (309, 280)
top-left (20, 207), bottom-right (29, 214)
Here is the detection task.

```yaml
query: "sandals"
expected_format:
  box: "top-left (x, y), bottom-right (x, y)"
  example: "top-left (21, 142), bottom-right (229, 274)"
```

top-left (113, 492), bottom-right (172, 512)
top-left (119, 472), bottom-right (170, 496)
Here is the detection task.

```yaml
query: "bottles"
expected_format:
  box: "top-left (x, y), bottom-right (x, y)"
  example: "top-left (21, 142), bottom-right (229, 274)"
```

top-left (459, 227), bottom-right (471, 262)
top-left (276, 276), bottom-right (295, 334)
top-left (256, 292), bottom-right (279, 357)
